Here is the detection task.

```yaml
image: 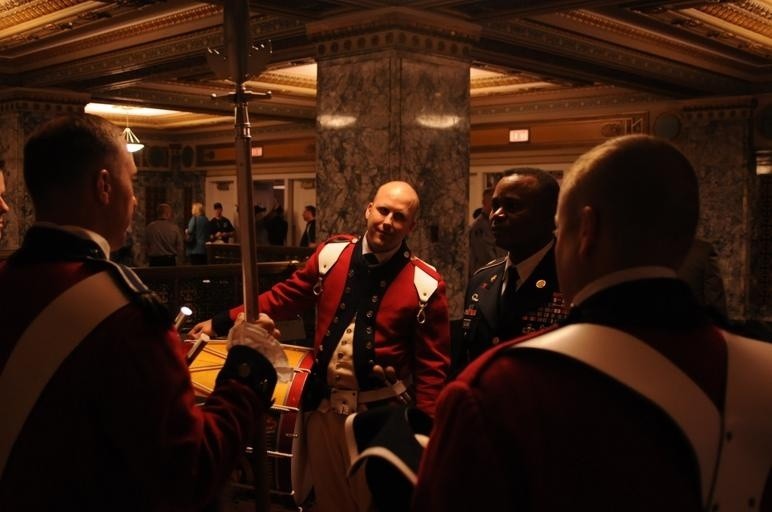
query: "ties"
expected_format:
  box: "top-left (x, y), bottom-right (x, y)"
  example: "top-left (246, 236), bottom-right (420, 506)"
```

top-left (498, 266), bottom-right (518, 314)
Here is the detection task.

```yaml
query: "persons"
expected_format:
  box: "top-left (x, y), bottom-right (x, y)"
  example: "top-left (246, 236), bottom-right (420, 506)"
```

top-left (143, 194), bottom-right (316, 272)
top-left (421, 130), bottom-right (772, 512)
top-left (0, 160), bottom-right (9, 241)
top-left (470, 207), bottom-right (493, 275)
top-left (0, 116), bottom-right (283, 512)
top-left (456, 168), bottom-right (569, 371)
top-left (186, 180), bottom-right (451, 511)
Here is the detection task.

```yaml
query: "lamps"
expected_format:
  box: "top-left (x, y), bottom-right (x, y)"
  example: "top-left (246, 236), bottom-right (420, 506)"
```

top-left (116, 105), bottom-right (144, 154)
